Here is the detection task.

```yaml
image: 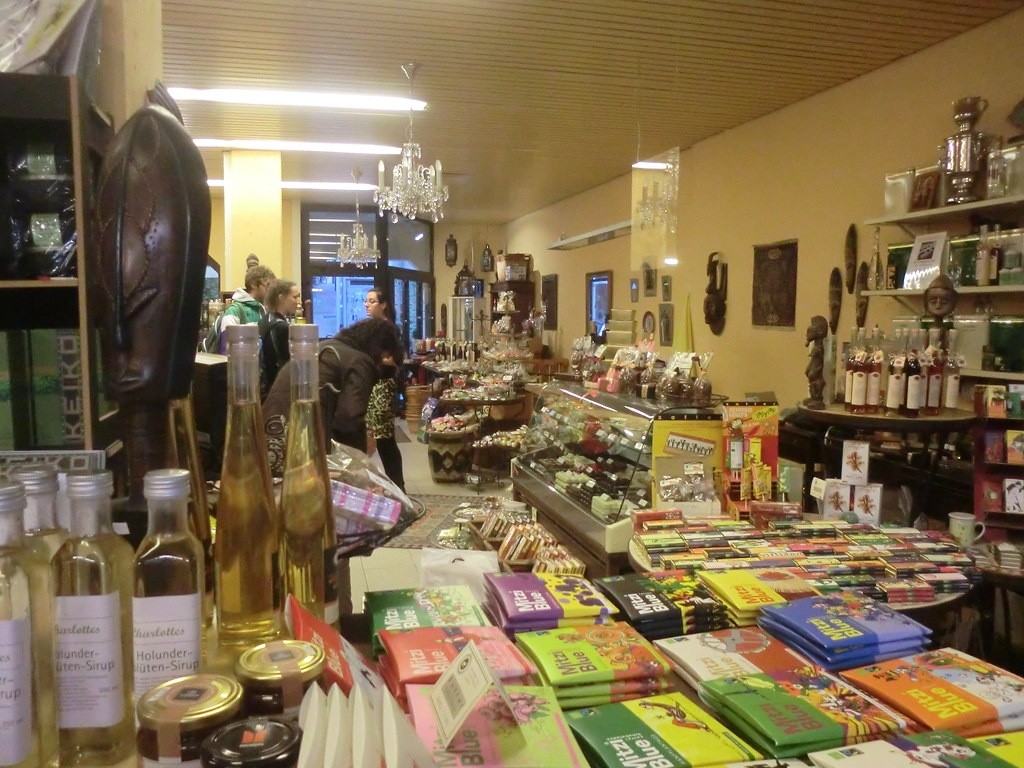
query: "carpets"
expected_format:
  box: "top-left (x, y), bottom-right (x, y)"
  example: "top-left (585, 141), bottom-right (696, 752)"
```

top-left (382, 494), bottom-right (485, 549)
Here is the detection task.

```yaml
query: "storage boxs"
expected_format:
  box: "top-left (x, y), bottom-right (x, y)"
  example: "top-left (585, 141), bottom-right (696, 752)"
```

top-left (495, 254), bottom-right (529, 281)
top-left (825, 439), bottom-right (882, 525)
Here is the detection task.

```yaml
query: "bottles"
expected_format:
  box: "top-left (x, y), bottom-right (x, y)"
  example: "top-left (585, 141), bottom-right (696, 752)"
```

top-left (974, 224), bottom-right (1003, 286)
top-left (927, 434), bottom-right (939, 467)
top-left (218, 318), bottom-right (335, 648)
top-left (843, 327), bottom-right (882, 415)
top-left (987, 135), bottom-right (1006, 198)
top-left (887, 325), bottom-right (959, 415)
top-left (413, 338), bottom-right (478, 363)
top-left (202, 298), bottom-right (231, 322)
top-left (867, 228), bottom-right (884, 290)
top-left (304, 296), bottom-right (313, 323)
top-left (133, 468), bottom-right (206, 730)
top-left (2, 463), bottom-right (133, 764)
top-left (295, 302), bottom-right (302, 323)
top-left (981, 346), bottom-right (997, 372)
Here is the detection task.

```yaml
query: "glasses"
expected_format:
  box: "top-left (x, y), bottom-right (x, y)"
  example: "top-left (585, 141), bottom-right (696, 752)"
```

top-left (364, 298), bottom-right (381, 304)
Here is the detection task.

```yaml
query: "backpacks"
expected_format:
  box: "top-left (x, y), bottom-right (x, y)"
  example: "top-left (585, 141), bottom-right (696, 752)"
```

top-left (196, 301), bottom-right (247, 355)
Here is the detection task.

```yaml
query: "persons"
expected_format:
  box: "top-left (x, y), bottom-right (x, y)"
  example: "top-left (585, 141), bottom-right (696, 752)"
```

top-left (361, 287), bottom-right (407, 494)
top-left (202, 266), bottom-right (396, 480)
top-left (923, 274), bottom-right (958, 350)
top-left (802, 317), bottom-right (829, 409)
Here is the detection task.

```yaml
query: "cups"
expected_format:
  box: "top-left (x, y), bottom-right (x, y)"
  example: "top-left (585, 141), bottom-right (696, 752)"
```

top-left (948, 513), bottom-right (986, 547)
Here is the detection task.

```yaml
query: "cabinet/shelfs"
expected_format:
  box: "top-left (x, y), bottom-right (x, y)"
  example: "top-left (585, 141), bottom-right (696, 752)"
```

top-left (0, 68), bottom-right (127, 467)
top-left (488, 282), bottom-right (533, 338)
top-left (858, 193), bottom-right (1024, 383)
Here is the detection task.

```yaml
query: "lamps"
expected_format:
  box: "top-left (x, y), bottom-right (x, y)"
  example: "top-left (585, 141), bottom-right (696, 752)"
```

top-left (373, 63), bottom-right (449, 223)
top-left (336, 168), bottom-right (381, 270)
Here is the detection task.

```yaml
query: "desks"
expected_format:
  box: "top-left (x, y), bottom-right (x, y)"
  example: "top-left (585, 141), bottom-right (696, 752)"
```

top-left (493, 442), bottom-right (516, 489)
top-left (799, 403), bottom-right (978, 528)
top-left (421, 363), bottom-right (475, 399)
top-left (437, 393), bottom-right (525, 496)
top-left (971, 543), bottom-right (1024, 675)
top-left (627, 538), bottom-right (976, 614)
